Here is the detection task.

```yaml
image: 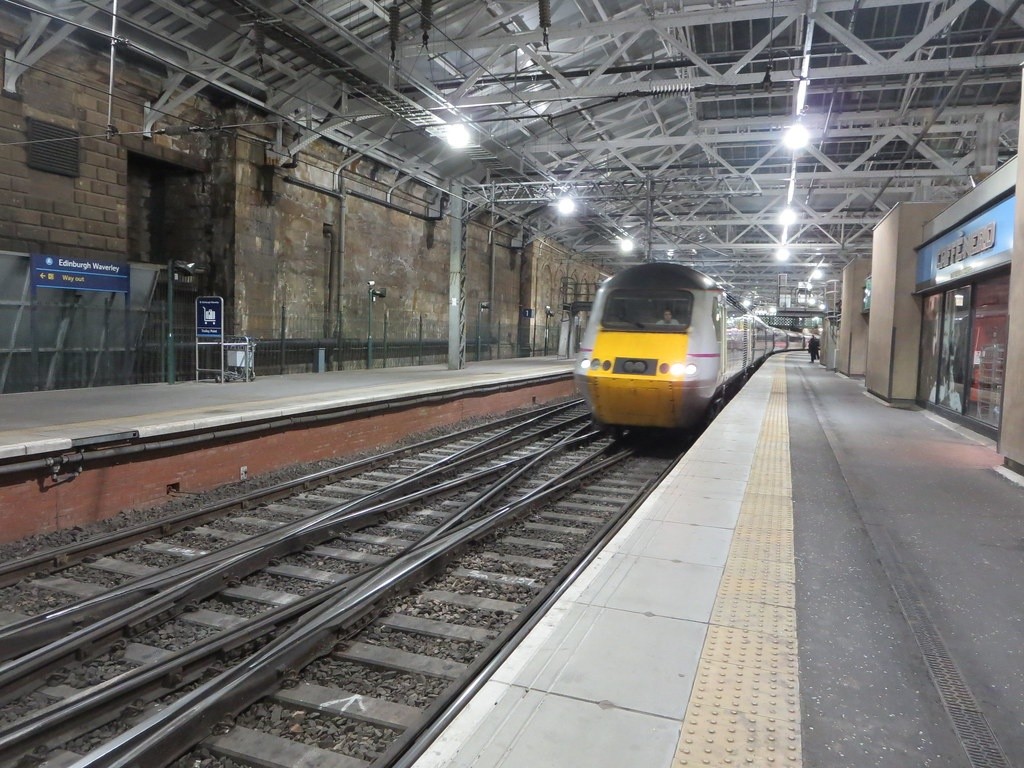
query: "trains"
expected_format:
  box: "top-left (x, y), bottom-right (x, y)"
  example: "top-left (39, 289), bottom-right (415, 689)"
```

top-left (573, 261), bottom-right (820, 436)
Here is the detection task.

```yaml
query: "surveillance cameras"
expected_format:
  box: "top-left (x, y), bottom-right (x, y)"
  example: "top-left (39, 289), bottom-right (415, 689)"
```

top-left (367, 281), bottom-right (375, 285)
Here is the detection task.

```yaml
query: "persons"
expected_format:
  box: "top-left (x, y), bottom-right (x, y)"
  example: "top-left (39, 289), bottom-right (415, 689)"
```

top-left (807, 335), bottom-right (820, 363)
top-left (656, 310), bottom-right (679, 326)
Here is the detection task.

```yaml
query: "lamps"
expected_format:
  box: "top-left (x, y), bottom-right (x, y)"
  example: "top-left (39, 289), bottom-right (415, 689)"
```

top-left (546, 311), bottom-right (555, 318)
top-left (372, 288), bottom-right (386, 301)
